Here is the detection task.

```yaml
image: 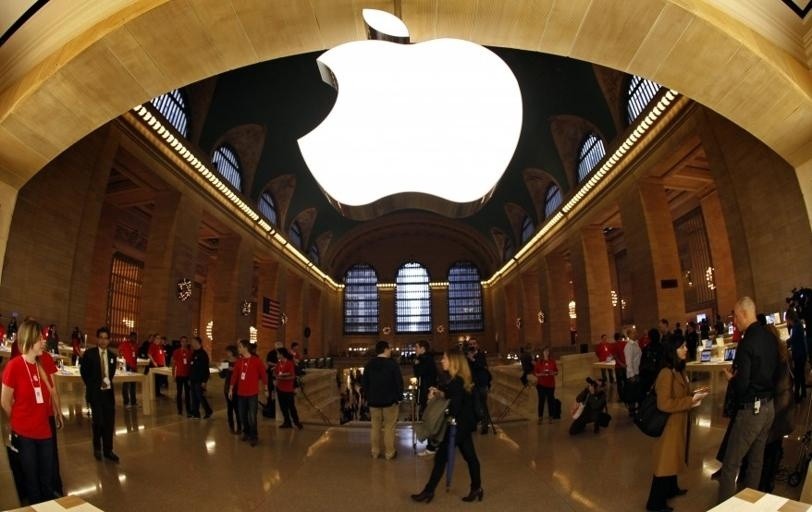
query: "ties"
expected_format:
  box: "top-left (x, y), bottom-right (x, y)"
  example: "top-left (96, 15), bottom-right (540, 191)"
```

top-left (100, 352), bottom-right (108, 388)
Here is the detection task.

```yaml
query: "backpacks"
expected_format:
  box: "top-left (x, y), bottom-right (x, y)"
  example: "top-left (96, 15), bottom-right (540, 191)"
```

top-left (628, 364), bottom-right (675, 439)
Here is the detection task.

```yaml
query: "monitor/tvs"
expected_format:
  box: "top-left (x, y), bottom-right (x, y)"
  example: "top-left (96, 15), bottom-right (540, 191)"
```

top-left (770, 312), bottom-right (782, 325)
top-left (701, 350), bottom-right (711, 362)
top-left (724, 348), bottom-right (737, 361)
top-left (696, 313), bottom-right (707, 323)
top-left (765, 315), bottom-right (773, 325)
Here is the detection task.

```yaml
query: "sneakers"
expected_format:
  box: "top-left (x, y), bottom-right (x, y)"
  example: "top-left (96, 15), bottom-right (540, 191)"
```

top-left (536, 415), bottom-right (553, 424)
top-left (368, 448), bottom-right (436, 461)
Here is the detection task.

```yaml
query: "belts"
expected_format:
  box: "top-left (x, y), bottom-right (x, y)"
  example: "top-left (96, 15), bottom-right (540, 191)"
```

top-left (740, 395), bottom-right (773, 409)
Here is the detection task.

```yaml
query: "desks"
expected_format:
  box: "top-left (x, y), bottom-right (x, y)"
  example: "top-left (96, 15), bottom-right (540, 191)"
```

top-left (3, 494), bottom-right (106, 512)
top-left (0, 330), bottom-right (220, 422)
top-left (594, 318), bottom-right (791, 413)
top-left (706, 487), bottom-right (812, 511)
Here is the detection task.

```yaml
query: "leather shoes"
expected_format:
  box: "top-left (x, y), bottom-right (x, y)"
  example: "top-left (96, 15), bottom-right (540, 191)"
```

top-left (646, 507), bottom-right (675, 512)
top-left (176, 410), bottom-right (303, 447)
top-left (667, 487), bottom-right (688, 498)
top-left (94, 450), bottom-right (102, 460)
top-left (104, 452), bottom-right (119, 462)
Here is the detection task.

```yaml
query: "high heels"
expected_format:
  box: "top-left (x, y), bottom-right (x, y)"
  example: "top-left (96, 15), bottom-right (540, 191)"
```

top-left (462, 487), bottom-right (483, 502)
top-left (411, 488), bottom-right (435, 503)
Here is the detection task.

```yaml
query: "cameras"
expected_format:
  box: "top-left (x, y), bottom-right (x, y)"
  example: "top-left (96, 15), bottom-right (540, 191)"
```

top-left (584, 375), bottom-right (598, 389)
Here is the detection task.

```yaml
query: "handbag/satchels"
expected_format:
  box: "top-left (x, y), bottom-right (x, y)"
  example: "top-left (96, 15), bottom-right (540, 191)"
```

top-left (569, 392), bottom-right (591, 420)
top-left (553, 392), bottom-right (562, 419)
top-left (722, 376), bottom-right (742, 417)
top-left (599, 401), bottom-right (612, 427)
